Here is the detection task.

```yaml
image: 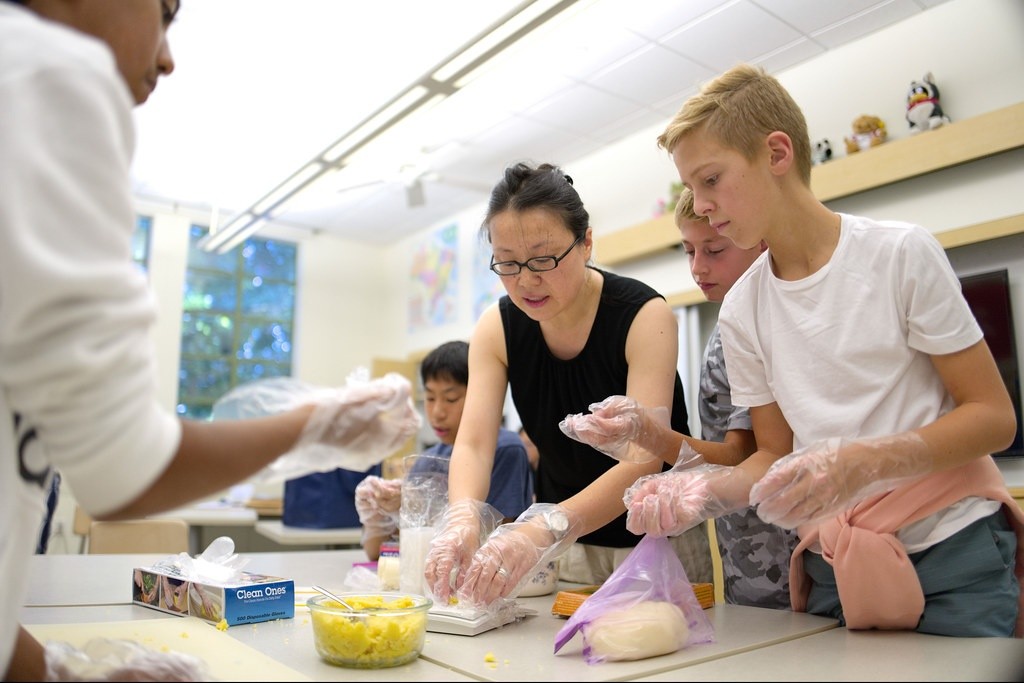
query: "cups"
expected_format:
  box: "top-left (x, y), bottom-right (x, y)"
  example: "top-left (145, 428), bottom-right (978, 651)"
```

top-left (398, 454), bottom-right (452, 594)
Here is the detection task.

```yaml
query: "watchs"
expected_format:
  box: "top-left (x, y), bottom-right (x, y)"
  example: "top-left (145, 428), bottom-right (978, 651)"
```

top-left (542, 508), bottom-right (569, 545)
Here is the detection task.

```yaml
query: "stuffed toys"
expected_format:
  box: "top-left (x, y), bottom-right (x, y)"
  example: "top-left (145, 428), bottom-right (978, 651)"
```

top-left (844, 116), bottom-right (886, 154)
top-left (906, 72), bottom-right (951, 134)
top-left (810, 139), bottom-right (831, 164)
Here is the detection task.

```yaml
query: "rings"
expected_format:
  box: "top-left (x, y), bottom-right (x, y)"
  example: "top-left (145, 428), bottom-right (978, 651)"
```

top-left (498, 569), bottom-right (508, 577)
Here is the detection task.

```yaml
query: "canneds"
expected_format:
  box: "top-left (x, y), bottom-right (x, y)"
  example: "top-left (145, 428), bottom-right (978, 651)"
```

top-left (377, 548), bottom-right (399, 589)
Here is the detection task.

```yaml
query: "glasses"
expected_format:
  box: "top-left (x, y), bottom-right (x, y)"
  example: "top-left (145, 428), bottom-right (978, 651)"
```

top-left (491, 233), bottom-right (580, 276)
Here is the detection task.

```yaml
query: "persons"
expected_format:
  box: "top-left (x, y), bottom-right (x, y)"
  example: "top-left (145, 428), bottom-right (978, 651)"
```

top-left (133, 570), bottom-right (158, 604)
top-left (518, 427), bottom-right (539, 502)
top-left (575, 186), bottom-right (799, 610)
top-left (193, 583), bottom-right (218, 618)
top-left (162, 576), bottom-right (188, 610)
top-left (355, 341), bottom-right (533, 561)
top-left (626, 68), bottom-right (1020, 636)
top-left (0, 0), bottom-right (417, 683)
top-left (424, 160), bottom-right (713, 609)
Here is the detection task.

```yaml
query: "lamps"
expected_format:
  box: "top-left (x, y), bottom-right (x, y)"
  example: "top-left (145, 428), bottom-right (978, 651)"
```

top-left (197, 1), bottom-right (578, 258)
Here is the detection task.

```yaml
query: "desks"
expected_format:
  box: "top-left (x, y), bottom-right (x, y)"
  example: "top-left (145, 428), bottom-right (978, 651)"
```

top-left (0, 505), bottom-right (1024, 682)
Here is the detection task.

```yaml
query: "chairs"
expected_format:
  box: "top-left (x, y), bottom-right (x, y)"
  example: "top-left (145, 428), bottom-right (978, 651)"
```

top-left (89, 520), bottom-right (189, 557)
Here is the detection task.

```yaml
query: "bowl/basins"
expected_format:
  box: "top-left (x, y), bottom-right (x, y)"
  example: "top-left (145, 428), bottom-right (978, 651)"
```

top-left (307, 592), bottom-right (433, 669)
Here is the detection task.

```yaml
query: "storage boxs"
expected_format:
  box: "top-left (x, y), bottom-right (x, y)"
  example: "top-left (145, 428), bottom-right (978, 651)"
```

top-left (130, 565), bottom-right (295, 626)
